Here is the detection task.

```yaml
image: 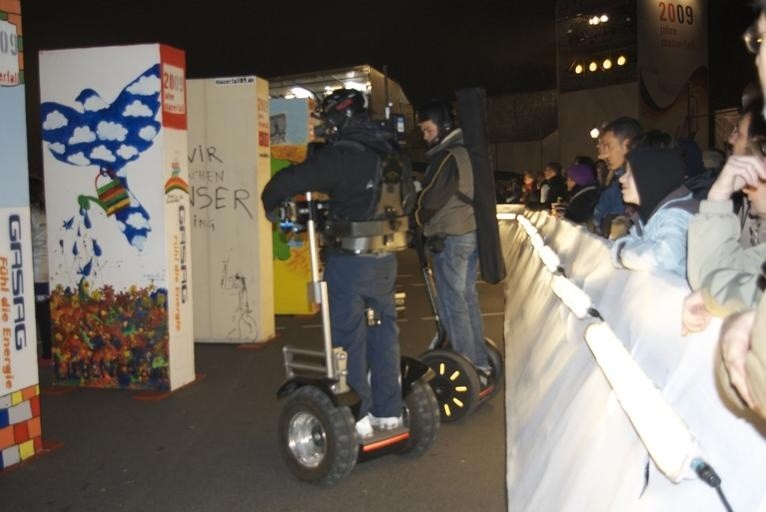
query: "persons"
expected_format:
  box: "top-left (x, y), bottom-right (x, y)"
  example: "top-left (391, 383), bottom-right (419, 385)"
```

top-left (498, 2), bottom-right (766, 428)
top-left (260, 89), bottom-right (418, 439)
top-left (415, 105), bottom-right (493, 394)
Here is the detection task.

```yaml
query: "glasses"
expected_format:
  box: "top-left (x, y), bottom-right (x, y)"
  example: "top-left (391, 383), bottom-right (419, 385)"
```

top-left (744, 26), bottom-right (766, 54)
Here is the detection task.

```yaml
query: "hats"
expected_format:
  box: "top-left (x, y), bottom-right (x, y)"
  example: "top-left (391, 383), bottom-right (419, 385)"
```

top-left (566, 165), bottom-right (594, 186)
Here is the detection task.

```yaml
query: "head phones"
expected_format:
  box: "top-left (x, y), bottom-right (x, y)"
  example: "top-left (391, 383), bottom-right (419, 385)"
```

top-left (438, 100), bottom-right (456, 137)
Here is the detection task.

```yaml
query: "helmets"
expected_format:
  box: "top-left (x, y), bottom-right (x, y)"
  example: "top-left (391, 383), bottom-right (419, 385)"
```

top-left (323, 89), bottom-right (364, 129)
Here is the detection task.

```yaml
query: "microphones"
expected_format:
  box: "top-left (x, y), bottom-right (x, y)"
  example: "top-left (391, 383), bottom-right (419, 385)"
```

top-left (423, 133), bottom-right (443, 150)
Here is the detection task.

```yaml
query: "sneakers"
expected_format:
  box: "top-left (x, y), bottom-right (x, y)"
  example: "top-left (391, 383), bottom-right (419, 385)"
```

top-left (368, 413), bottom-right (401, 433)
top-left (475, 369), bottom-right (496, 399)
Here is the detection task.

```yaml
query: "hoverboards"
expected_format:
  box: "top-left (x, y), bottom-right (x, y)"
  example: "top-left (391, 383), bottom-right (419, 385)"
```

top-left (380, 181), bottom-right (502, 426)
top-left (274, 185), bottom-right (439, 492)
